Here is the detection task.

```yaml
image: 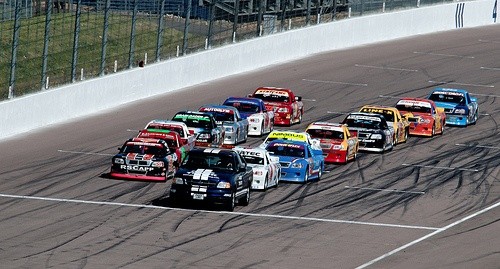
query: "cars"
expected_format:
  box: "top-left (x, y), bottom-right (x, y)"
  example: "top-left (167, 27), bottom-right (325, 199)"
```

top-left (223, 96), bottom-right (275, 137)
top-left (249, 86), bottom-right (305, 127)
top-left (394, 96), bottom-right (447, 137)
top-left (198, 103), bottom-right (250, 146)
top-left (259, 129), bottom-right (326, 184)
top-left (339, 112), bottom-right (393, 154)
top-left (172, 109), bottom-right (226, 148)
top-left (426, 87), bottom-right (480, 127)
top-left (358, 105), bottom-right (411, 145)
top-left (305, 122), bottom-right (359, 166)
top-left (232, 145), bottom-right (282, 191)
top-left (111, 119), bottom-right (196, 182)
top-left (169, 147), bottom-right (253, 211)
